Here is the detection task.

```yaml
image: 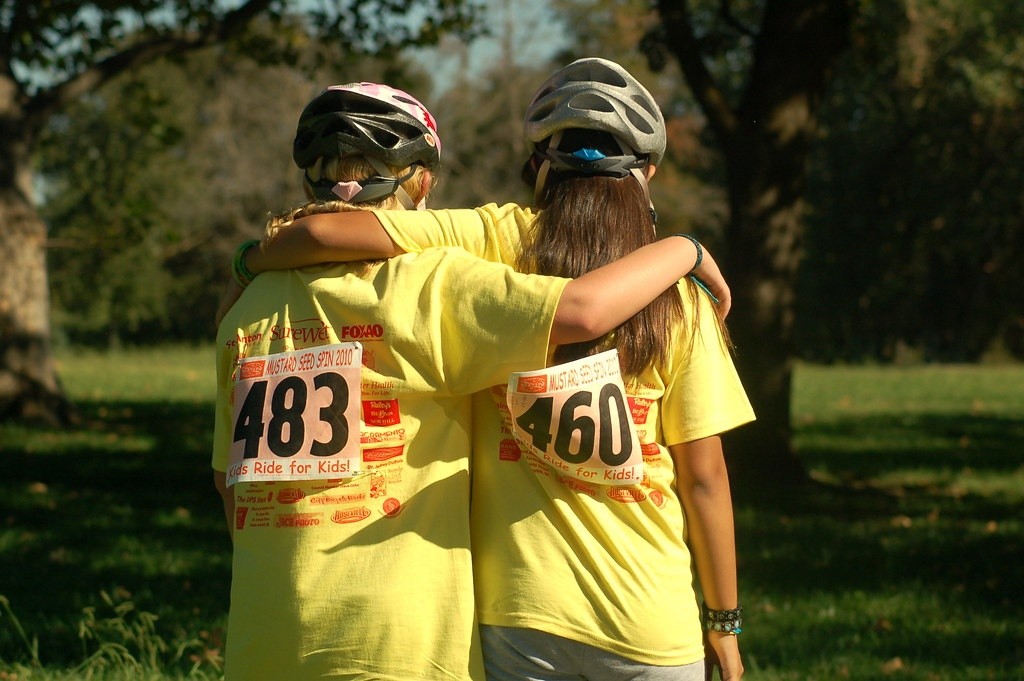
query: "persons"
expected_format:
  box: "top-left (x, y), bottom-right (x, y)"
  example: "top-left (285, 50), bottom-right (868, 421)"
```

top-left (212, 79), bottom-right (733, 681)
top-left (212, 57), bottom-right (746, 681)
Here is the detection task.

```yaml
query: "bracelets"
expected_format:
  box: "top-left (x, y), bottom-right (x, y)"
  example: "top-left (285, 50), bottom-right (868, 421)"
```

top-left (670, 232), bottom-right (703, 270)
top-left (232, 238), bottom-right (261, 289)
top-left (702, 600), bottom-right (744, 634)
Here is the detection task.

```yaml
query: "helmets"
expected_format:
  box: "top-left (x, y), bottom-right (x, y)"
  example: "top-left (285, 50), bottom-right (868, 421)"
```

top-left (523, 57), bottom-right (667, 176)
top-left (293, 82), bottom-right (441, 171)
